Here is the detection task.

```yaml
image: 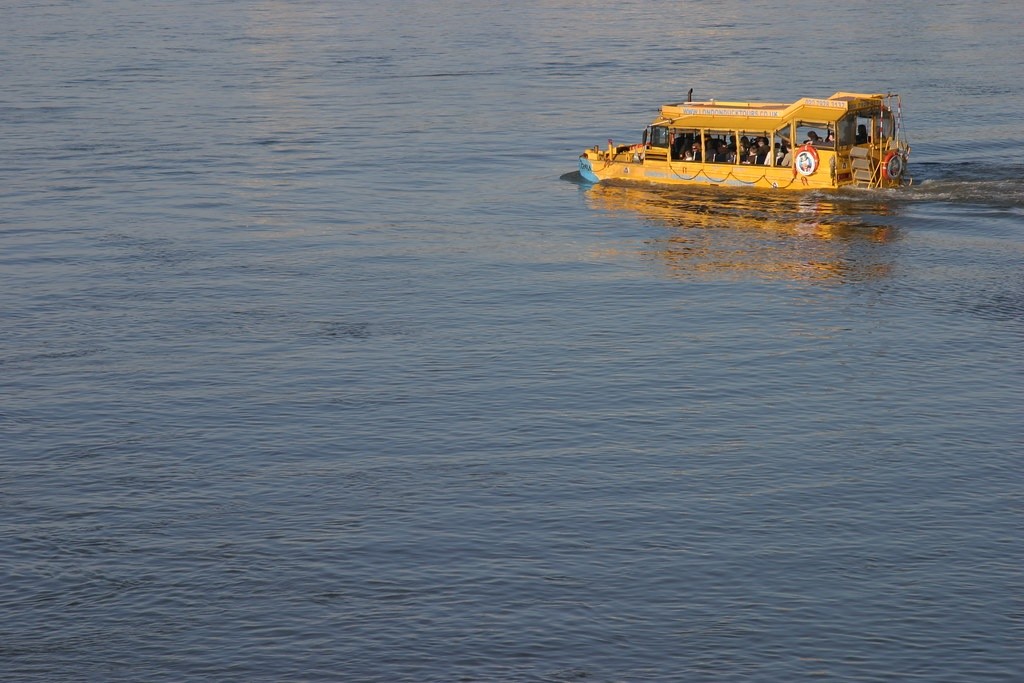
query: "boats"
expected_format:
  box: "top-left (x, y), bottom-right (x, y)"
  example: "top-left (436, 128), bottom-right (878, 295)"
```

top-left (578, 88), bottom-right (911, 190)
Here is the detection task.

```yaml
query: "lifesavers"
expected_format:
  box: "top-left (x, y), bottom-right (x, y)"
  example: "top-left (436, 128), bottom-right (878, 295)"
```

top-left (883, 152), bottom-right (902, 180)
top-left (795, 144), bottom-right (819, 176)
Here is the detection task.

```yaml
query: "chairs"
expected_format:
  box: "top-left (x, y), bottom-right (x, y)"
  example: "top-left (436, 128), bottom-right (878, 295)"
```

top-left (713, 153), bottom-right (719, 162)
top-left (755, 152), bottom-right (767, 164)
top-left (694, 152), bottom-right (702, 161)
top-left (776, 157), bottom-right (783, 165)
top-left (734, 155), bottom-right (748, 164)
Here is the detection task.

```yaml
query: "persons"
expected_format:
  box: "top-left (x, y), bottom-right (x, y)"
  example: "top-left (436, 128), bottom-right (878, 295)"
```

top-left (856, 125), bottom-right (886, 144)
top-left (673, 131), bottom-right (834, 166)
